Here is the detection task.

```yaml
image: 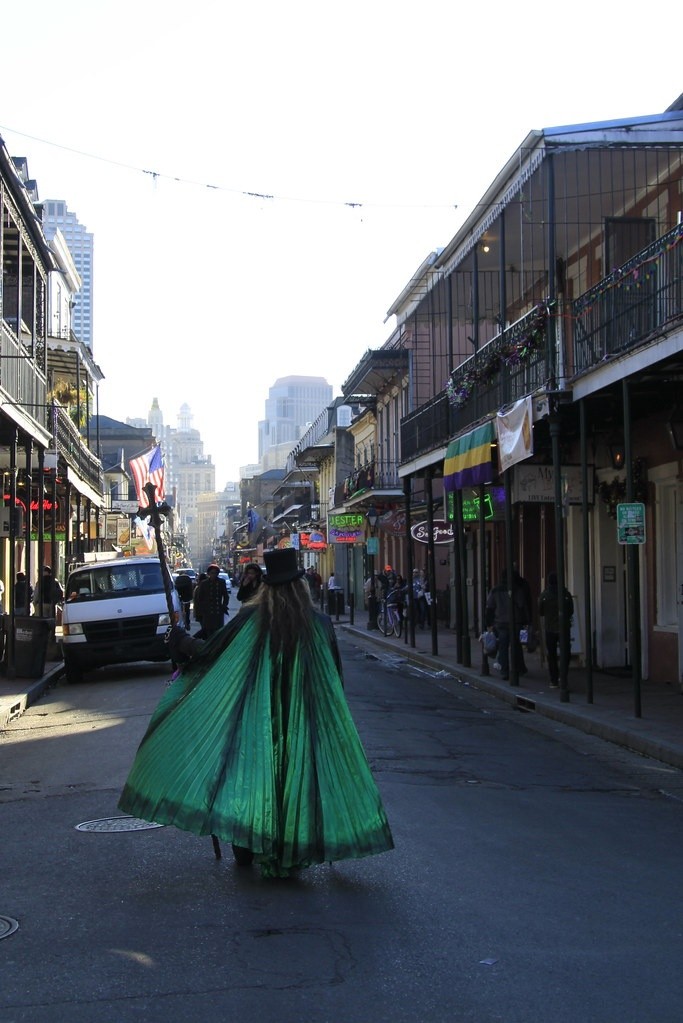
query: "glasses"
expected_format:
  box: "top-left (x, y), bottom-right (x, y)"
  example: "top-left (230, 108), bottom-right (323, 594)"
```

top-left (210, 572), bottom-right (217, 575)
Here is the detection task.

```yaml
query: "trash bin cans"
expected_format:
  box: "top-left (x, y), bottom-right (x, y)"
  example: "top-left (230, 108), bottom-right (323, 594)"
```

top-left (328, 586), bottom-right (345, 621)
top-left (4, 616), bottom-right (56, 680)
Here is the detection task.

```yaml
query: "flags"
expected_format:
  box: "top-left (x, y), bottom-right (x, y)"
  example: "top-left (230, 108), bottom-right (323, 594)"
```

top-left (130, 443), bottom-right (164, 505)
top-left (248, 510), bottom-right (259, 533)
top-left (134, 514), bottom-right (163, 550)
top-left (443, 420), bottom-right (493, 493)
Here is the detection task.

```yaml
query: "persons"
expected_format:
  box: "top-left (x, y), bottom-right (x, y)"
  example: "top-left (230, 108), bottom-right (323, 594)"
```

top-left (119, 549), bottom-right (395, 881)
top-left (175, 570), bottom-right (193, 627)
top-left (237, 563), bottom-right (263, 603)
top-left (193, 563), bottom-right (229, 639)
top-left (328, 573), bottom-right (334, 589)
top-left (304, 566), bottom-right (321, 602)
top-left (14, 573), bottom-right (34, 616)
top-left (486, 568), bottom-right (574, 687)
top-left (34, 565), bottom-right (63, 618)
top-left (363, 564), bottom-right (431, 629)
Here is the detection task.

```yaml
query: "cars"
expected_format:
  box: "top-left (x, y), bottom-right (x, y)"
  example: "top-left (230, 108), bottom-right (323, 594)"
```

top-left (169, 568), bottom-right (199, 590)
top-left (218, 573), bottom-right (234, 594)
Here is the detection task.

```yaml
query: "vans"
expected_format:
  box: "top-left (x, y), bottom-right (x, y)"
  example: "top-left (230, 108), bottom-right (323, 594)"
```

top-left (41, 553), bottom-right (194, 683)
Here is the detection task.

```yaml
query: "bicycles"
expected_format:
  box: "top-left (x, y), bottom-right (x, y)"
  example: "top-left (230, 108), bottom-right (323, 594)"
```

top-left (377, 589), bottom-right (402, 638)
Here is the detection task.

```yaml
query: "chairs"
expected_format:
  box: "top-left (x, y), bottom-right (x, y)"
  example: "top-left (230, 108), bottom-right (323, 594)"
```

top-left (142, 575), bottom-right (159, 589)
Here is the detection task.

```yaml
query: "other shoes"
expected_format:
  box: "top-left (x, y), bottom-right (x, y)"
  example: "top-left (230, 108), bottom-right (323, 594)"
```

top-left (549, 682), bottom-right (559, 689)
top-left (232, 844), bottom-right (254, 864)
top-left (502, 674), bottom-right (509, 679)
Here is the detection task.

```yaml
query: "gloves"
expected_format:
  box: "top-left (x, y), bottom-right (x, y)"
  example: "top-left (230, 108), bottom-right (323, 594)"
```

top-left (164, 626), bottom-right (179, 645)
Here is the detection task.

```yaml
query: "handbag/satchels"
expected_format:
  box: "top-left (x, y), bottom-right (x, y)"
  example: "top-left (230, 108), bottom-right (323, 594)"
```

top-left (425, 592), bottom-right (437, 608)
top-left (518, 627), bottom-right (529, 644)
top-left (478, 630), bottom-right (497, 659)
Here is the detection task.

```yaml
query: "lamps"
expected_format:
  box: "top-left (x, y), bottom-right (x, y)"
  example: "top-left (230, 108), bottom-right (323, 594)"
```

top-left (606, 428), bottom-right (625, 471)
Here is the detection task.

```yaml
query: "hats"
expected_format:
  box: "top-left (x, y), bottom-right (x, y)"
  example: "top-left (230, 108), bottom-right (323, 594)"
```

top-left (385, 565), bottom-right (392, 571)
top-left (261, 548), bottom-right (305, 584)
top-left (44, 566), bottom-right (51, 572)
top-left (207, 564), bottom-right (220, 573)
top-left (16, 573), bottom-right (25, 581)
